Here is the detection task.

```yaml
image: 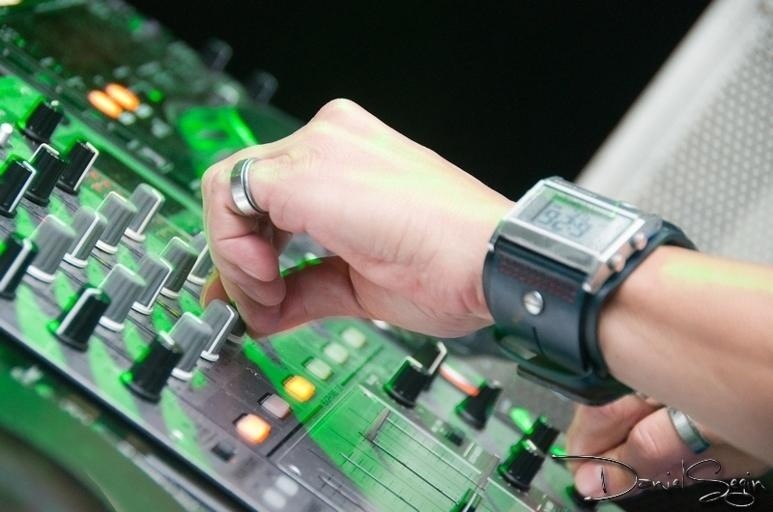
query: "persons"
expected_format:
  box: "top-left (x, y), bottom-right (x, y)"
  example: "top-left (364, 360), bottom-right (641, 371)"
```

top-left (201, 98), bottom-right (773, 501)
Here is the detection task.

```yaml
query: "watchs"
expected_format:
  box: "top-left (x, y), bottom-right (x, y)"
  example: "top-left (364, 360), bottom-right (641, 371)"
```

top-left (483, 176), bottom-right (700, 407)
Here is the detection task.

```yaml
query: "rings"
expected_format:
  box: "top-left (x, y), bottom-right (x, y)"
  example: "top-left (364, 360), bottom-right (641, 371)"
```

top-left (667, 407), bottom-right (711, 453)
top-left (230, 158), bottom-right (267, 220)
